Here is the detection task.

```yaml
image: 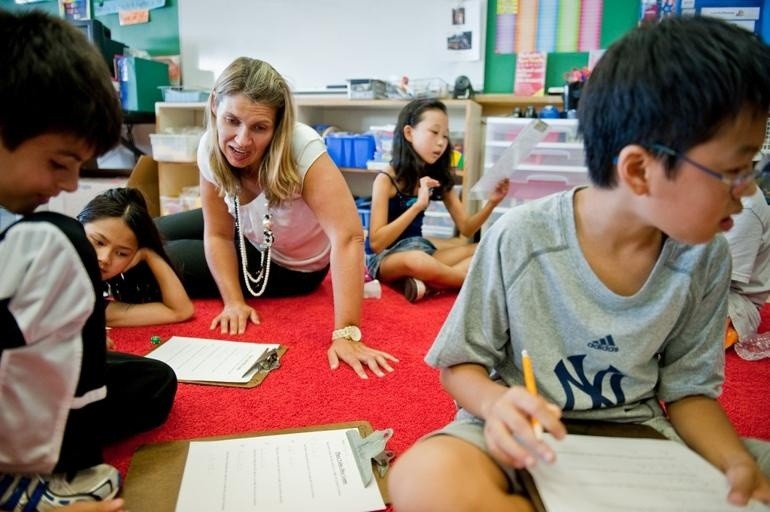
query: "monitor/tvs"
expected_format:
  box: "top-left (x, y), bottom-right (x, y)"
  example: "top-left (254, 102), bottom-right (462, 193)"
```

top-left (72, 19), bottom-right (129, 79)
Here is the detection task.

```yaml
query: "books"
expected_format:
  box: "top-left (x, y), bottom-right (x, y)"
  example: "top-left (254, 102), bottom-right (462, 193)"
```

top-left (513, 50), bottom-right (548, 98)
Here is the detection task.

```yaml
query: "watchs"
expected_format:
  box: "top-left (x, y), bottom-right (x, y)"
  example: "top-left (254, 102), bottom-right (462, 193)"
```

top-left (329, 324), bottom-right (362, 343)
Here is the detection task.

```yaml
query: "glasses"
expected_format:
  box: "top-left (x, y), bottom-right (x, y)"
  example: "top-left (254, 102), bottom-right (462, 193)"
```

top-left (612, 143), bottom-right (769, 191)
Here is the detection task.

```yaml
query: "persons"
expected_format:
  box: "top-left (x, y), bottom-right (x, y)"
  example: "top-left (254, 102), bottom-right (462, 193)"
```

top-left (123, 56), bottom-right (402, 381)
top-left (386, 10), bottom-right (769, 512)
top-left (364, 97), bottom-right (510, 303)
top-left (718, 180), bottom-right (770, 351)
top-left (0, 9), bottom-right (177, 511)
top-left (78, 186), bottom-right (196, 351)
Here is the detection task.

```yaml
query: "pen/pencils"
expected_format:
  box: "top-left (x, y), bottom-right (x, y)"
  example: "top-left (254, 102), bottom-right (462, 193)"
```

top-left (326, 85), bottom-right (347, 89)
top-left (520, 348), bottom-right (541, 440)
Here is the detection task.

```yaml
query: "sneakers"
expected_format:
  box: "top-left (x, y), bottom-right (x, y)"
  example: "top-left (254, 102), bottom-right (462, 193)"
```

top-left (0, 463), bottom-right (121, 512)
top-left (404, 278), bottom-right (426, 302)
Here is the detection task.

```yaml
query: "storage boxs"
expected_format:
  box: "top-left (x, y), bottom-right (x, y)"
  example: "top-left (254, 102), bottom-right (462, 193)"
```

top-left (313, 125), bottom-right (463, 239)
top-left (482, 117), bottom-right (593, 240)
top-left (150, 134), bottom-right (203, 163)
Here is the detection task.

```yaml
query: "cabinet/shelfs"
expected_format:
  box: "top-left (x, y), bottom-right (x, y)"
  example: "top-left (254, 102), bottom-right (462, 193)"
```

top-left (154, 99), bottom-right (482, 243)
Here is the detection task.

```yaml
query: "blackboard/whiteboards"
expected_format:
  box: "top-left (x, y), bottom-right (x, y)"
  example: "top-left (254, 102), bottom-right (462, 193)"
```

top-left (175, 1), bottom-right (489, 95)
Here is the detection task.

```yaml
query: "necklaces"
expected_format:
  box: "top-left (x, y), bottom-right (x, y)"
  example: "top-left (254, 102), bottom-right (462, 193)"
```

top-left (232, 176), bottom-right (275, 298)
